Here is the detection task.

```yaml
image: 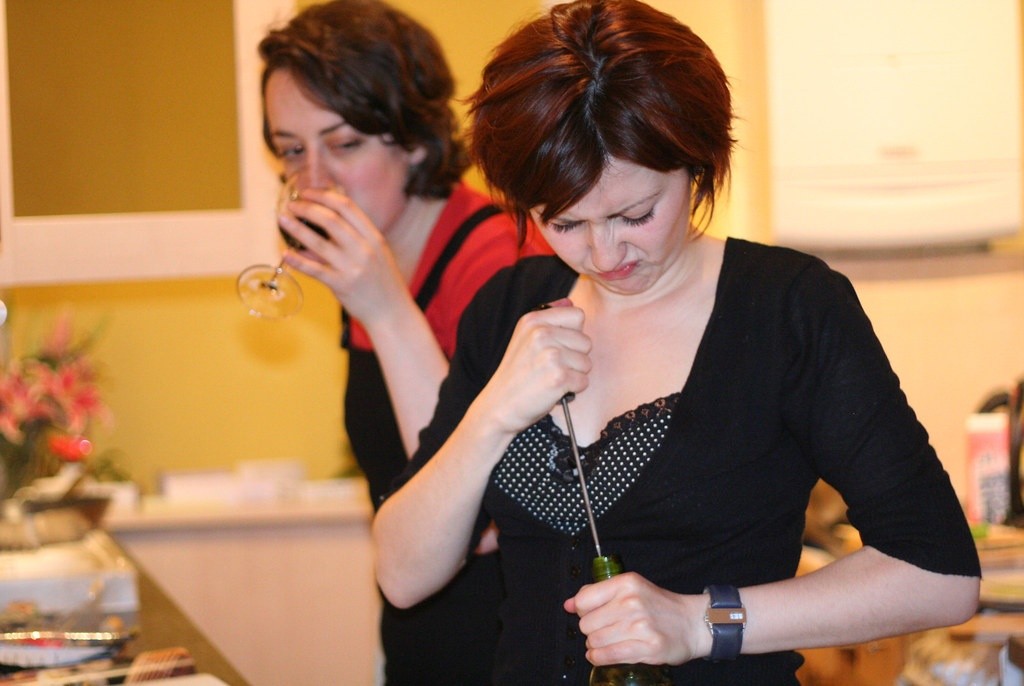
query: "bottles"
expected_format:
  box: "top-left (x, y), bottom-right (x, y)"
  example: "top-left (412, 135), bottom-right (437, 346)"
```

top-left (590, 554), bottom-right (675, 686)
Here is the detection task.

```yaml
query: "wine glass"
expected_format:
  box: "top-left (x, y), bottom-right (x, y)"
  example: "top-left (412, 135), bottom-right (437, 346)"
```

top-left (237, 170), bottom-right (347, 320)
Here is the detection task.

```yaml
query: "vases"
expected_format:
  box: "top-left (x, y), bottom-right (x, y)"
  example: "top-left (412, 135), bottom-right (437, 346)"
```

top-left (20, 489), bottom-right (110, 527)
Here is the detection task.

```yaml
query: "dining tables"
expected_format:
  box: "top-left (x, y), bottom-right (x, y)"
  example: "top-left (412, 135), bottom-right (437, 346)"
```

top-left (0, 526), bottom-right (245, 686)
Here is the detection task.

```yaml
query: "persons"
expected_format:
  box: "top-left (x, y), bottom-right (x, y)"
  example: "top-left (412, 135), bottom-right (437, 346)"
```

top-left (260, 3), bottom-right (587, 686)
top-left (369, 0), bottom-right (984, 686)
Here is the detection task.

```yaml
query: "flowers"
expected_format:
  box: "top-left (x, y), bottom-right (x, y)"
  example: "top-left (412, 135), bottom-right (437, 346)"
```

top-left (0, 306), bottom-right (106, 502)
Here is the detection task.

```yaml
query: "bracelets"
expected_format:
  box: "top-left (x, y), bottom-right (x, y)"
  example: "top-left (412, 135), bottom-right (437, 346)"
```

top-left (705, 583), bottom-right (747, 661)
